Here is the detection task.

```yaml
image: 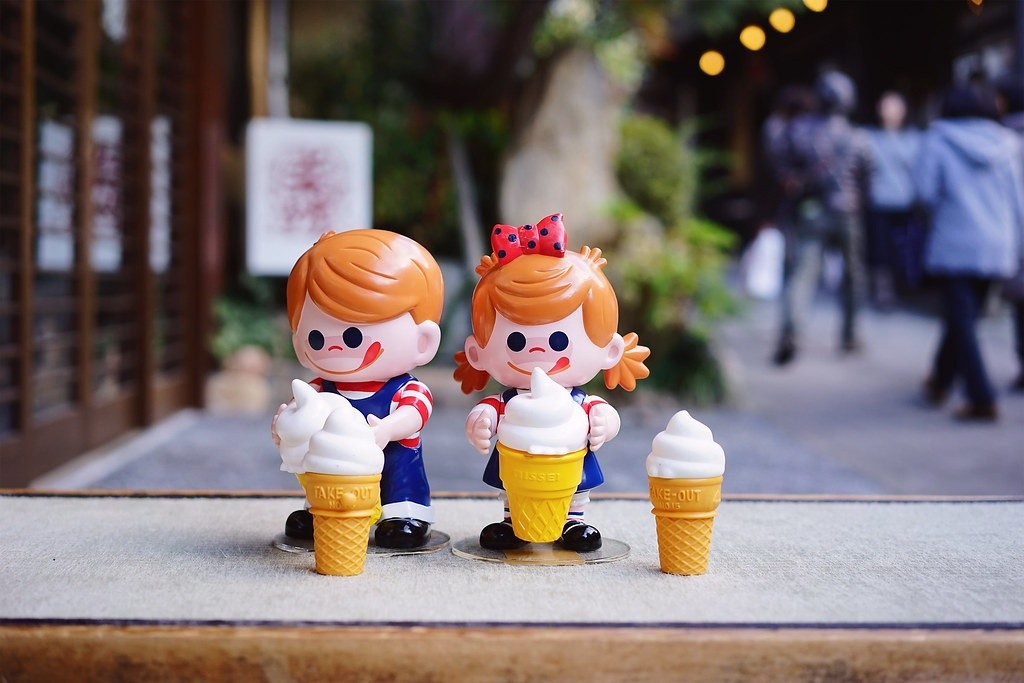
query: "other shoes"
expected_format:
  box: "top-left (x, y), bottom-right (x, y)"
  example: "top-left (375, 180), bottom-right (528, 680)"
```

top-left (962, 408), bottom-right (996, 421)
top-left (774, 335), bottom-right (794, 364)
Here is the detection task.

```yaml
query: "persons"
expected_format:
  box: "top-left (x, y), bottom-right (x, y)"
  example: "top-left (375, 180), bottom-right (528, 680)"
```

top-left (455, 246), bottom-right (650, 555)
top-left (741, 45), bottom-right (1023, 423)
top-left (270, 227), bottom-right (444, 550)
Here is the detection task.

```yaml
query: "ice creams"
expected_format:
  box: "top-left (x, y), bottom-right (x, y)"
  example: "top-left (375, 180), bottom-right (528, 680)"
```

top-left (496, 367), bottom-right (590, 542)
top-left (646, 410), bottom-right (726, 576)
top-left (276, 379), bottom-right (384, 577)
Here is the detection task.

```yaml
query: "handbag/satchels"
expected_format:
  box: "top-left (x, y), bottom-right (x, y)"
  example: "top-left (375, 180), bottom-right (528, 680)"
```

top-left (742, 229), bottom-right (787, 300)
